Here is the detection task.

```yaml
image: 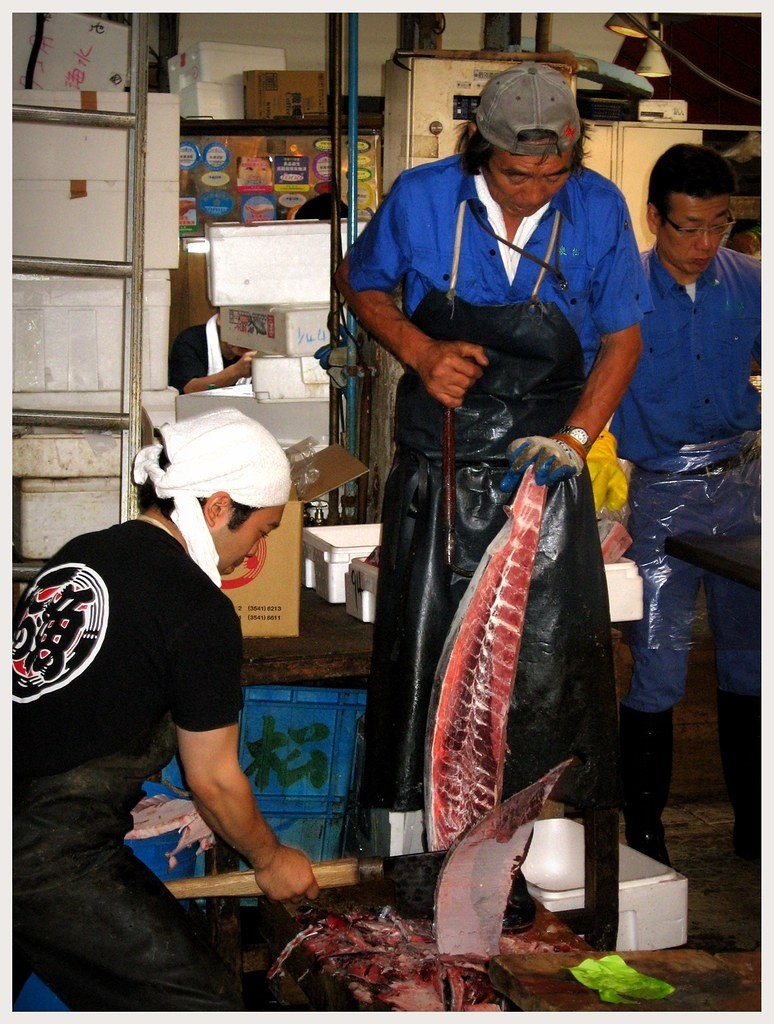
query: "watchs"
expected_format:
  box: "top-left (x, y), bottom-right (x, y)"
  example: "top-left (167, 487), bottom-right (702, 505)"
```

top-left (560, 425), bottom-right (591, 452)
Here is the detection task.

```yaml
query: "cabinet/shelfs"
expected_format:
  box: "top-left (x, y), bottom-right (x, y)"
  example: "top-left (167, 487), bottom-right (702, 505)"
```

top-left (12, 13), bottom-right (147, 590)
top-left (582, 121), bottom-right (703, 253)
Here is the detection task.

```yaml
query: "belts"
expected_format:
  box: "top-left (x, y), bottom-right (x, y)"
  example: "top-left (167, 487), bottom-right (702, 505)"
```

top-left (631, 447), bottom-right (760, 475)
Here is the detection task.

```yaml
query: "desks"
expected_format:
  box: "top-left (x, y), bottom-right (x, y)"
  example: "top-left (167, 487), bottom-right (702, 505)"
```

top-left (664, 532), bottom-right (760, 593)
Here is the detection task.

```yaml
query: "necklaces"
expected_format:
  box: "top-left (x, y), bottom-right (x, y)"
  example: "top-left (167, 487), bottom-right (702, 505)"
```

top-left (467, 199), bottom-right (570, 291)
top-left (136, 513), bottom-right (174, 540)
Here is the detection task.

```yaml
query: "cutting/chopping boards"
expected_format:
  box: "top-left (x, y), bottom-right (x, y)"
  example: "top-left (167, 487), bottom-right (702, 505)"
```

top-left (489, 949), bottom-right (761, 1012)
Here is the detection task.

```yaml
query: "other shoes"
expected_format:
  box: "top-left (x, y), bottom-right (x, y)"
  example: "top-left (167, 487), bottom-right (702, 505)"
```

top-left (500, 868), bottom-right (536, 934)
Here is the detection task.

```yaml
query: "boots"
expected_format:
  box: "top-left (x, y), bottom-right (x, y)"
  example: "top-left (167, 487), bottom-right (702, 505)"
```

top-left (618, 700), bottom-right (673, 872)
top-left (714, 683), bottom-right (761, 866)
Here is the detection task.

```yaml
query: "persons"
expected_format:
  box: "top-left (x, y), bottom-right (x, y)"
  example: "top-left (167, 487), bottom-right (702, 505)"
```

top-left (333, 63), bottom-right (655, 935)
top-left (607, 143), bottom-right (761, 873)
top-left (12, 405), bottom-right (320, 1012)
top-left (168, 307), bottom-right (257, 395)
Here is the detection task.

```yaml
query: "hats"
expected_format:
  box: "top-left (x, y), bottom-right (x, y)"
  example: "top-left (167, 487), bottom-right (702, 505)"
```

top-left (475, 61), bottom-right (582, 158)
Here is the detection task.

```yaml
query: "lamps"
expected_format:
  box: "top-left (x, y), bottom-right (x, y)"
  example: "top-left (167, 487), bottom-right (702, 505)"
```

top-left (605, 14), bottom-right (761, 105)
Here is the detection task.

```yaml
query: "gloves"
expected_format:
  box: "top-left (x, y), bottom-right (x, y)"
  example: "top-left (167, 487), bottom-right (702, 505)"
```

top-left (499, 436), bottom-right (577, 493)
top-left (586, 428), bottom-right (628, 512)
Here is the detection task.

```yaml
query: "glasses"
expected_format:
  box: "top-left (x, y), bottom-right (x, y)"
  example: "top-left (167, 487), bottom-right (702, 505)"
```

top-left (657, 208), bottom-right (736, 239)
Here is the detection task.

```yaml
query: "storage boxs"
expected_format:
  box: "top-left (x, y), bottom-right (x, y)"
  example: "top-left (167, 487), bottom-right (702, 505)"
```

top-left (206, 219), bottom-right (366, 307)
top-left (242, 69), bottom-right (327, 119)
top-left (220, 305), bottom-right (333, 356)
top-left (173, 384), bottom-right (330, 447)
top-left (161, 685), bottom-right (365, 907)
top-left (220, 443), bottom-right (371, 638)
top-left (522, 818), bottom-right (689, 952)
top-left (13, 88), bottom-right (178, 559)
top-left (302, 521), bottom-right (644, 625)
top-left (251, 352), bottom-right (330, 403)
top-left (167, 41), bottom-right (287, 119)
